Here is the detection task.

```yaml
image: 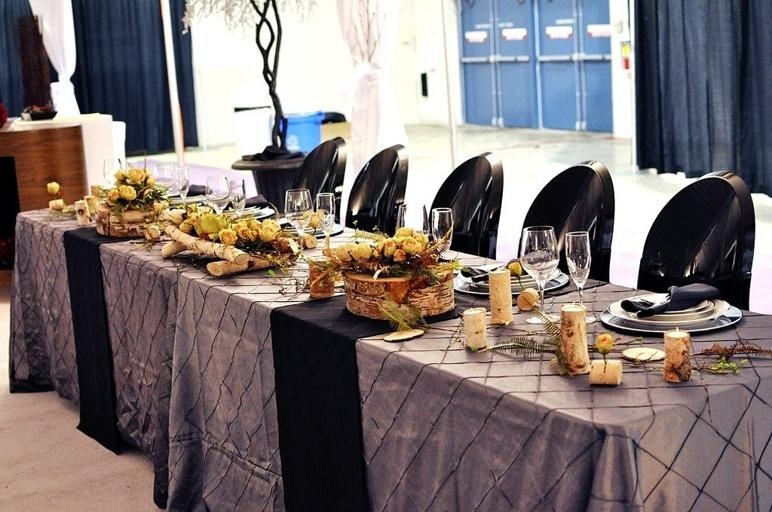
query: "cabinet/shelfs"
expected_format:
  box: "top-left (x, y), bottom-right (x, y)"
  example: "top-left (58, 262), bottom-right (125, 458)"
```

top-left (0, 114), bottom-right (105, 212)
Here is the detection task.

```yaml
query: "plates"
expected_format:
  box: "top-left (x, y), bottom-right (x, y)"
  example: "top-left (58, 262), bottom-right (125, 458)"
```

top-left (600, 305), bottom-right (743, 333)
top-left (455, 273), bottom-right (568, 295)
top-left (485, 278), bottom-right (535, 283)
top-left (664, 299), bottom-right (710, 314)
top-left (285, 224), bottom-right (343, 237)
top-left (511, 274), bottom-right (530, 279)
top-left (653, 299), bottom-right (717, 317)
top-left (224, 205), bottom-right (275, 219)
top-left (459, 265), bottom-right (561, 292)
top-left (606, 293), bottom-right (731, 326)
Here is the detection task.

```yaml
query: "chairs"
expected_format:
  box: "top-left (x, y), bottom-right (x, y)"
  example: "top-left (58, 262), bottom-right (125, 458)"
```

top-left (345, 143), bottom-right (408, 236)
top-left (636, 170), bottom-right (754, 311)
top-left (425, 151), bottom-right (503, 260)
top-left (516, 158), bottom-right (615, 282)
top-left (292, 136), bottom-right (346, 224)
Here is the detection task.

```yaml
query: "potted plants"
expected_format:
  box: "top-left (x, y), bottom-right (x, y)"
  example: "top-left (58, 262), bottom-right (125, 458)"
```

top-left (182, 0), bottom-right (312, 213)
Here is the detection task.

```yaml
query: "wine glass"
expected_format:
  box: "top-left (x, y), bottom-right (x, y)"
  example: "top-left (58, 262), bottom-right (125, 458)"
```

top-left (152, 164), bottom-right (174, 193)
top-left (316, 192), bottom-right (335, 248)
top-left (229, 176), bottom-right (246, 216)
top-left (175, 167), bottom-right (189, 205)
top-left (565, 231), bottom-right (591, 305)
top-left (205, 176), bottom-right (230, 216)
top-left (520, 226), bottom-right (561, 324)
top-left (396, 203), bottom-right (430, 238)
top-left (432, 208), bottom-right (453, 259)
top-left (103, 158), bottom-right (122, 187)
top-left (284, 189), bottom-right (314, 263)
